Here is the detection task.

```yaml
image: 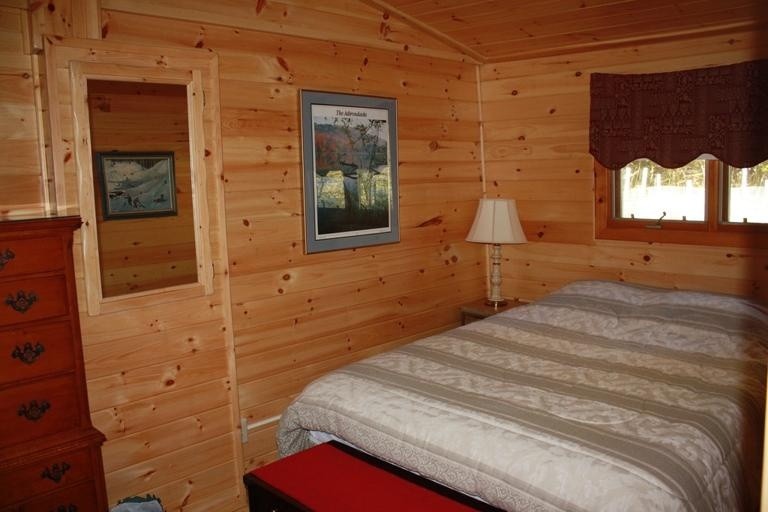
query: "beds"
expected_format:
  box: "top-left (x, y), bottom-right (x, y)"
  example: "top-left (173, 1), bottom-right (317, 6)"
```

top-left (275, 279), bottom-right (768, 512)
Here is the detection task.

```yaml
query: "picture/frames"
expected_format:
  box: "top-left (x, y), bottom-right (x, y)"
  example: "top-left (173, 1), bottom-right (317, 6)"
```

top-left (297, 87), bottom-right (402, 255)
top-left (95, 150), bottom-right (178, 220)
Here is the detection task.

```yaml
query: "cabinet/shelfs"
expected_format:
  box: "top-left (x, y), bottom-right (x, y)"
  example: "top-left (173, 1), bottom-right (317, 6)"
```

top-left (0, 218), bottom-right (106, 512)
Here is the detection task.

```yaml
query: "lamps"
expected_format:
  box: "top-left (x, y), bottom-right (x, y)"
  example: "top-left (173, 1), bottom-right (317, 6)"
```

top-left (465, 196), bottom-right (527, 306)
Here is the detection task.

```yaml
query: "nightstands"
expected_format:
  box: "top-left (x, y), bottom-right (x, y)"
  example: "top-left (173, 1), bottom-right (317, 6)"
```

top-left (460, 295), bottom-right (529, 326)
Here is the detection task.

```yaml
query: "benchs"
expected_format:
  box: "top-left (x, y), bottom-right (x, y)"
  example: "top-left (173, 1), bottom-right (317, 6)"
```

top-left (245, 440), bottom-right (496, 512)
